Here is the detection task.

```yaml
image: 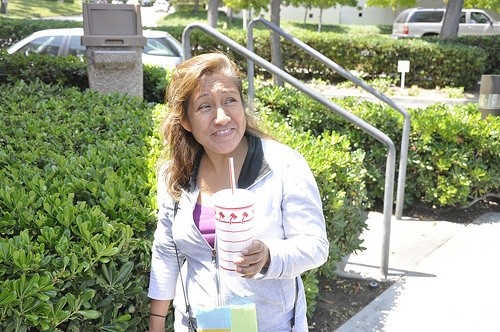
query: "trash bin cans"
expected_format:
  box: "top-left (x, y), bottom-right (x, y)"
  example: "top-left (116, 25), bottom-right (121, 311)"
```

top-left (478, 75), bottom-right (500, 118)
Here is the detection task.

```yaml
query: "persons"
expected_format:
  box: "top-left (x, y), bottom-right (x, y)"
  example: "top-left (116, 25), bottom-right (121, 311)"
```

top-left (147, 53), bottom-right (330, 332)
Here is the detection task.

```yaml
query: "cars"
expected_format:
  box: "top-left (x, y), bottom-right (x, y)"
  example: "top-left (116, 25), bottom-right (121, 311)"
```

top-left (392, 7), bottom-right (500, 39)
top-left (95, 0), bottom-right (171, 14)
top-left (6, 28), bottom-right (183, 75)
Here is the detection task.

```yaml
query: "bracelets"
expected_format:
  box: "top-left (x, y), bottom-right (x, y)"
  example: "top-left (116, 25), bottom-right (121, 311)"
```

top-left (149, 313), bottom-right (167, 318)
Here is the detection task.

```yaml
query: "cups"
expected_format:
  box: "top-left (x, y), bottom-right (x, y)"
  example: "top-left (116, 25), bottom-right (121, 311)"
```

top-left (213, 188), bottom-right (254, 276)
